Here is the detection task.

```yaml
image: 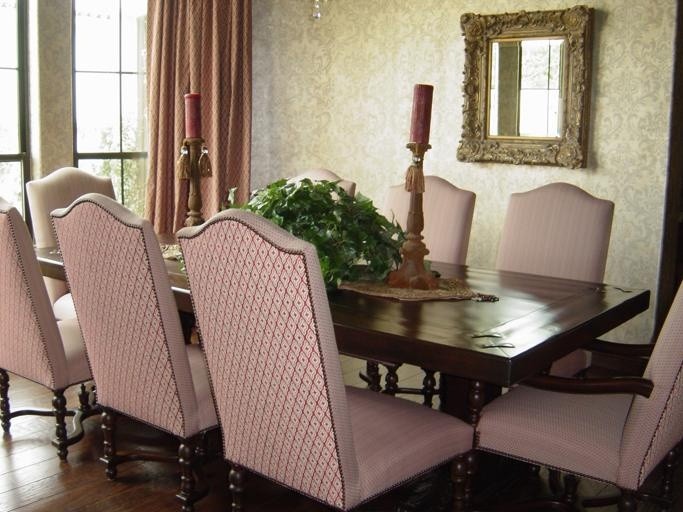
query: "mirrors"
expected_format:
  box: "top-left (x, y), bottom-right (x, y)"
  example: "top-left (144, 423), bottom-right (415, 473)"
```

top-left (456, 7), bottom-right (597, 170)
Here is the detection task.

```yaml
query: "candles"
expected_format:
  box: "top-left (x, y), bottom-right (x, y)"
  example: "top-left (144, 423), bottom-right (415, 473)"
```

top-left (409, 82), bottom-right (434, 144)
top-left (183, 91), bottom-right (201, 139)
